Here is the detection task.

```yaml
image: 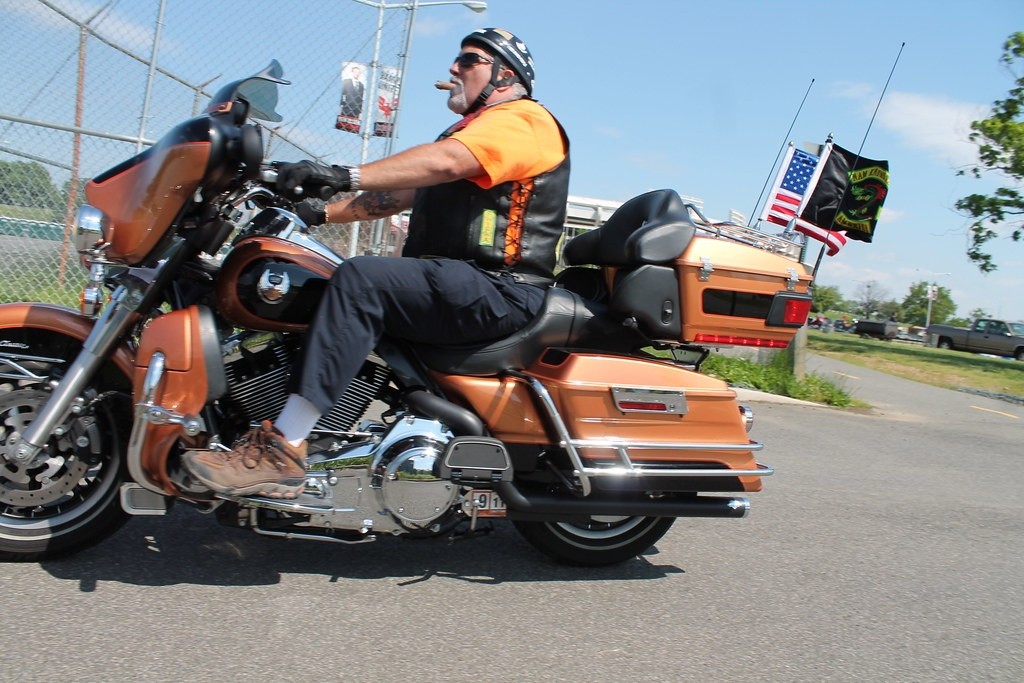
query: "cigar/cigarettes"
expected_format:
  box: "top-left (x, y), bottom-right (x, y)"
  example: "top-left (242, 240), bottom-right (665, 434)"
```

top-left (434, 80), bottom-right (456, 90)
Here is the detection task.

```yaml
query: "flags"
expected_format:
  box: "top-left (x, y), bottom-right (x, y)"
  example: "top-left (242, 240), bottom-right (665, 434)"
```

top-left (796, 141), bottom-right (890, 243)
top-left (760, 146), bottom-right (849, 255)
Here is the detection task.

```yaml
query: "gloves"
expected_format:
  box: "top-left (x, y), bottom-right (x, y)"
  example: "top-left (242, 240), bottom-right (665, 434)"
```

top-left (295, 198), bottom-right (326, 226)
top-left (271, 159), bottom-right (350, 202)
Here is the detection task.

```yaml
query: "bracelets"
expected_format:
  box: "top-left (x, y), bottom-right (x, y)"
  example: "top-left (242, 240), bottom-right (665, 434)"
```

top-left (323, 205), bottom-right (329, 222)
top-left (343, 163), bottom-right (360, 192)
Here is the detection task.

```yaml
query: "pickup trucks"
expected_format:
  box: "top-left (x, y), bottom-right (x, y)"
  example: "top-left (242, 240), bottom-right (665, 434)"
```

top-left (926, 317), bottom-right (1024, 361)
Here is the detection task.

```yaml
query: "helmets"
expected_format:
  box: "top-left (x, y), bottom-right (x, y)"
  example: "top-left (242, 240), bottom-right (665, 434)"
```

top-left (460, 27), bottom-right (536, 94)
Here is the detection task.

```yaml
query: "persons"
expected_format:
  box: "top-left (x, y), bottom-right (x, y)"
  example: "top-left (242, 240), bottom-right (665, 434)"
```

top-left (817, 315), bottom-right (824, 331)
top-left (185, 27), bottom-right (571, 498)
top-left (341, 67), bottom-right (364, 119)
top-left (890, 311), bottom-right (898, 322)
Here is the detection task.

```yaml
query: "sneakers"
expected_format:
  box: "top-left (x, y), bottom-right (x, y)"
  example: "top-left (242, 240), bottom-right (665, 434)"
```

top-left (181, 420), bottom-right (307, 500)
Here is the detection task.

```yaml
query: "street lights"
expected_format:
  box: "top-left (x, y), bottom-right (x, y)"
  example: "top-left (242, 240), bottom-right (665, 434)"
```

top-left (346, 1), bottom-right (490, 261)
top-left (917, 268), bottom-right (952, 327)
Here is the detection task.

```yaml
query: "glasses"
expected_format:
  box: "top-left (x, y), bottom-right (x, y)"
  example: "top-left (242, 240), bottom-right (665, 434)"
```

top-left (454, 52), bottom-right (506, 70)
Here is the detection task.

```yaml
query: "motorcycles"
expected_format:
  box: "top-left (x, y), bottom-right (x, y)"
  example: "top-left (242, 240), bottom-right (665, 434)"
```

top-left (0, 58), bottom-right (814, 567)
